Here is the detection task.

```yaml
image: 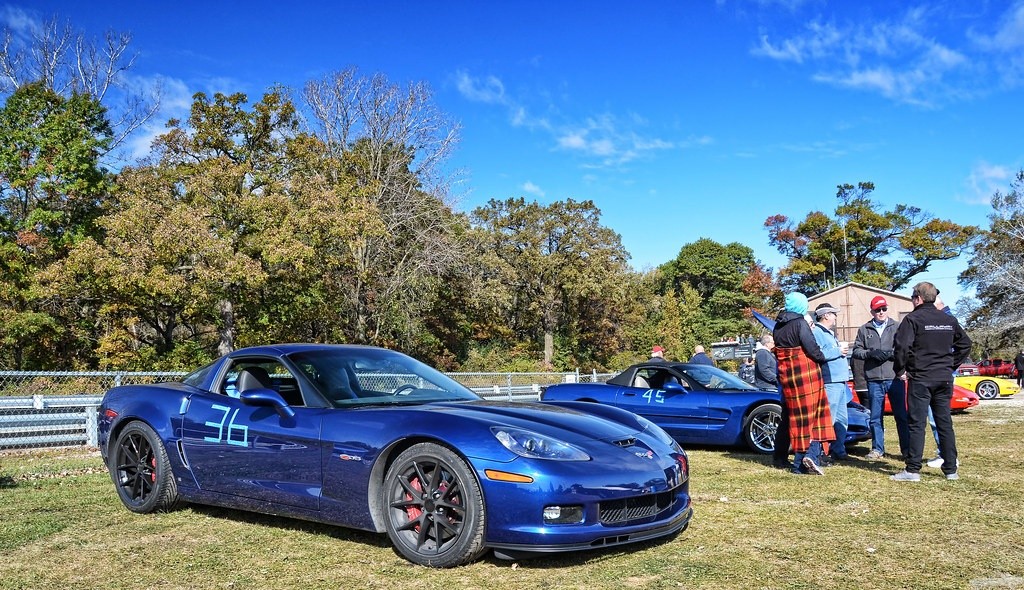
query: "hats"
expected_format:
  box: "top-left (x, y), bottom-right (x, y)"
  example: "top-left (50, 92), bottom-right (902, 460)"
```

top-left (814, 306), bottom-right (841, 317)
top-left (653, 346), bottom-right (665, 352)
top-left (870, 296), bottom-right (887, 310)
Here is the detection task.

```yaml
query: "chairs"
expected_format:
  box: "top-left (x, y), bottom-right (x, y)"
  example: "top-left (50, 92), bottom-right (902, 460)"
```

top-left (239, 367), bottom-right (272, 403)
top-left (315, 367), bottom-right (358, 401)
top-left (663, 376), bottom-right (678, 391)
top-left (634, 376), bottom-right (651, 388)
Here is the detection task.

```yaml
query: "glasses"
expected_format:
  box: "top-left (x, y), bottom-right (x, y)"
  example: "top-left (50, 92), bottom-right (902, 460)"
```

top-left (874, 307), bottom-right (888, 313)
top-left (911, 296), bottom-right (918, 298)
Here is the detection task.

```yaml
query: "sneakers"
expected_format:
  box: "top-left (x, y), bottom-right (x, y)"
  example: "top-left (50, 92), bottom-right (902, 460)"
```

top-left (927, 455), bottom-right (960, 469)
top-left (865, 450), bottom-right (885, 459)
top-left (946, 471), bottom-right (959, 480)
top-left (888, 470), bottom-right (920, 481)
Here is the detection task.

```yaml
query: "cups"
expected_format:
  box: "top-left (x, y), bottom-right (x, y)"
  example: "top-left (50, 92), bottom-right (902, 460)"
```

top-left (841, 342), bottom-right (848, 350)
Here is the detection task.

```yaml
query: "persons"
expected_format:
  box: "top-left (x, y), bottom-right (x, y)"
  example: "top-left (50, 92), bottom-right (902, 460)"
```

top-left (755, 335), bottom-right (776, 386)
top-left (849, 356), bottom-right (869, 409)
top-left (738, 357), bottom-right (755, 383)
top-left (647, 346), bottom-right (666, 377)
top-left (926, 296), bottom-right (960, 468)
top-left (686, 345), bottom-right (714, 384)
top-left (852, 296), bottom-right (909, 459)
top-left (889, 282), bottom-right (973, 482)
top-left (772, 291), bottom-right (836, 475)
top-left (812, 303), bottom-right (848, 466)
top-left (1014, 349), bottom-right (1024, 388)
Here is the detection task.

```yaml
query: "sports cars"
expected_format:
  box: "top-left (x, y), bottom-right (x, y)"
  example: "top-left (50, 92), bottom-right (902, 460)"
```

top-left (842, 354), bottom-right (981, 416)
top-left (711, 371), bottom-right (875, 451)
top-left (538, 356), bottom-right (786, 455)
top-left (96, 342), bottom-right (697, 566)
top-left (949, 357), bottom-right (1021, 400)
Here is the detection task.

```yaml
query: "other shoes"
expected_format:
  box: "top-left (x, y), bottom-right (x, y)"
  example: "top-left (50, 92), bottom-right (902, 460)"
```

top-left (819, 455), bottom-right (834, 466)
top-left (791, 465), bottom-right (806, 474)
top-left (803, 456), bottom-right (824, 476)
top-left (831, 454), bottom-right (859, 462)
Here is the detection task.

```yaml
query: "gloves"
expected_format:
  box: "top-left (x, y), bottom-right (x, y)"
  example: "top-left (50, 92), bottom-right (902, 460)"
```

top-left (866, 349), bottom-right (888, 361)
top-left (875, 350), bottom-right (892, 363)
